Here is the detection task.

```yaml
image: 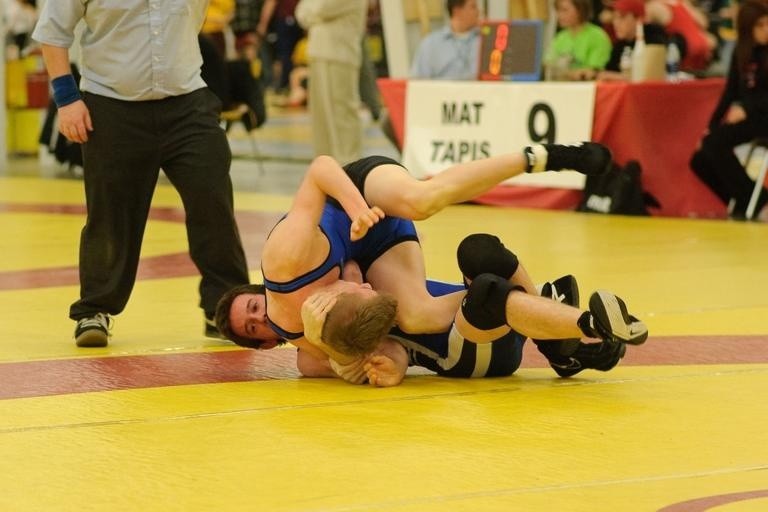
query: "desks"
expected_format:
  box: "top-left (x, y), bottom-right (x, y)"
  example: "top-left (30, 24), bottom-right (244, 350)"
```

top-left (401, 77), bottom-right (730, 220)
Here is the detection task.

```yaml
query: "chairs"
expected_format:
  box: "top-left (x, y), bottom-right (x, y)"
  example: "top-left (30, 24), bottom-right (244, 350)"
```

top-left (376, 79), bottom-right (406, 146)
top-left (220, 57), bottom-right (266, 175)
top-left (733, 144), bottom-right (768, 219)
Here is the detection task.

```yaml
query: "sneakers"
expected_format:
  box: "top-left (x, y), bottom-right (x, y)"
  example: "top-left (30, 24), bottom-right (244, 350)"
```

top-left (205, 313), bottom-right (229, 340)
top-left (549, 342), bottom-right (625, 378)
top-left (74, 316), bottom-right (112, 349)
top-left (546, 273), bottom-right (580, 312)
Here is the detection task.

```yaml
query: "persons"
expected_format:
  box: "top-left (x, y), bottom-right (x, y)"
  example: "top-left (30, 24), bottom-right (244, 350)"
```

top-left (31, 0), bottom-right (252, 350)
top-left (255, 138), bottom-right (614, 388)
top-left (211, 232), bottom-right (652, 385)
top-left (685, 0), bottom-right (768, 222)
top-left (1, 0), bottom-right (83, 174)
top-left (201, 1), bottom-right (737, 180)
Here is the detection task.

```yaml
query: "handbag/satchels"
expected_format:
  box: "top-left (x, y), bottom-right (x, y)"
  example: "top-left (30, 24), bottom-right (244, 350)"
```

top-left (575, 157), bottom-right (656, 217)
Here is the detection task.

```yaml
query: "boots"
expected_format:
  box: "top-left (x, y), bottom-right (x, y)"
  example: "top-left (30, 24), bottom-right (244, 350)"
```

top-left (525, 138), bottom-right (611, 183)
top-left (577, 285), bottom-right (650, 345)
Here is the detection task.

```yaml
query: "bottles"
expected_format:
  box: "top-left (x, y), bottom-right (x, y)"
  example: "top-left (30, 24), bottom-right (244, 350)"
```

top-left (620, 46), bottom-right (632, 81)
top-left (665, 38), bottom-right (680, 82)
top-left (633, 20), bottom-right (648, 82)
